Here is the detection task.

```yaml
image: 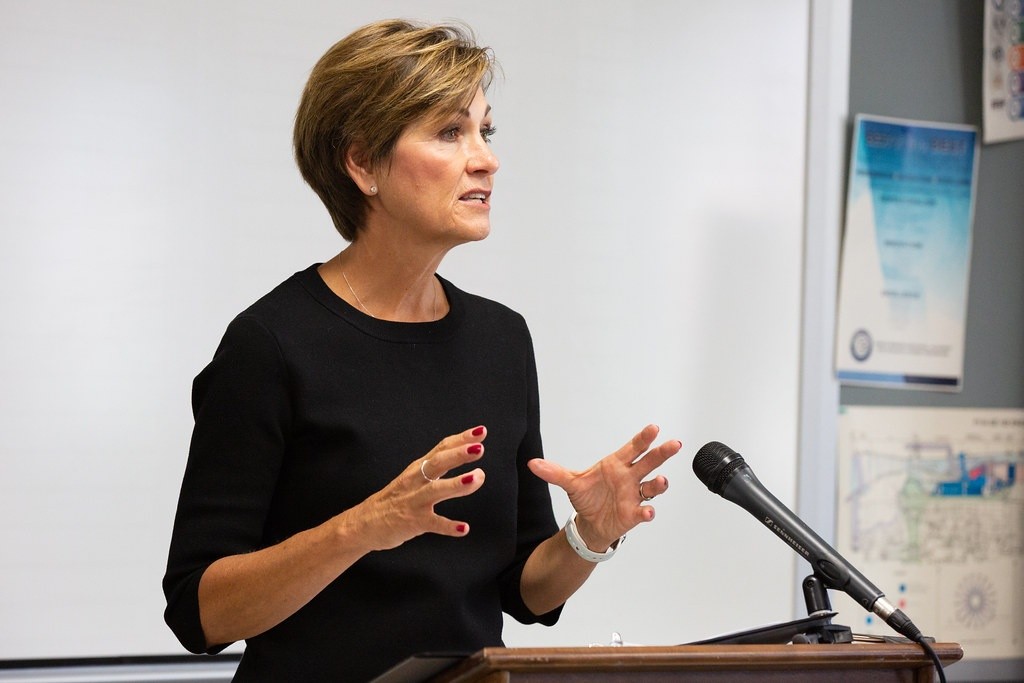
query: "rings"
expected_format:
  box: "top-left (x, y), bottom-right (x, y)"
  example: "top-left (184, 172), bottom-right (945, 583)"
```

top-left (421, 460), bottom-right (440, 482)
top-left (640, 481), bottom-right (655, 501)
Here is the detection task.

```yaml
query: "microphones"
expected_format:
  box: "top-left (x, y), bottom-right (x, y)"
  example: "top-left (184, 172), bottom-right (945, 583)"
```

top-left (693, 441), bottom-right (924, 642)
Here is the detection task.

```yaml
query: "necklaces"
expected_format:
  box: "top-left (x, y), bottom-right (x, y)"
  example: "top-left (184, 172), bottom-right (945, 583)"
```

top-left (338, 252), bottom-right (438, 321)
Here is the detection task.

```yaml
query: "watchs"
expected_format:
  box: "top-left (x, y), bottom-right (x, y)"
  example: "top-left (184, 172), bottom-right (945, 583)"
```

top-left (564, 510), bottom-right (626, 563)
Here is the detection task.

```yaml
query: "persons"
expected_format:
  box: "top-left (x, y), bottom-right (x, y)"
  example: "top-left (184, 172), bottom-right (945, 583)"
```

top-left (162, 25), bottom-right (681, 683)
